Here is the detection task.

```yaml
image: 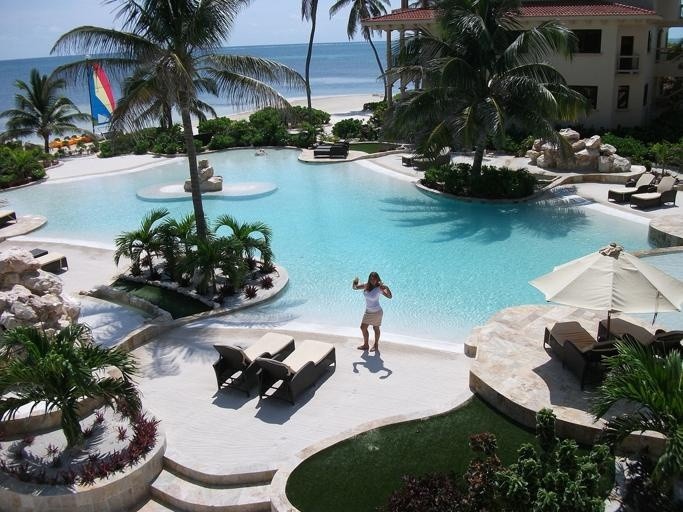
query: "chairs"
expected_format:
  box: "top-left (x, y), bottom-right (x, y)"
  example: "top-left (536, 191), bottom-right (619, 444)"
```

top-left (314, 142), bottom-right (349, 158)
top-left (256, 339), bottom-right (336, 406)
top-left (608, 173), bottom-right (656, 204)
top-left (0, 210), bottom-right (17, 227)
top-left (37, 253), bottom-right (68, 273)
top-left (630, 176), bottom-right (677, 210)
top-left (544, 321), bottom-right (620, 390)
top-left (597, 318), bottom-right (683, 351)
top-left (402, 144), bottom-right (452, 170)
top-left (29, 248), bottom-right (48, 258)
top-left (212, 333), bottom-right (295, 398)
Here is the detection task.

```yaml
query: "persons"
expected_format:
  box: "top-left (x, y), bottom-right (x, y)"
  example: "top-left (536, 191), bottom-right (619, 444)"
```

top-left (351, 271), bottom-right (392, 353)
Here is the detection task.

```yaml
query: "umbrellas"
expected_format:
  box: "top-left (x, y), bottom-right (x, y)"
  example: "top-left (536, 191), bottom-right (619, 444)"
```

top-left (528, 242), bottom-right (683, 342)
